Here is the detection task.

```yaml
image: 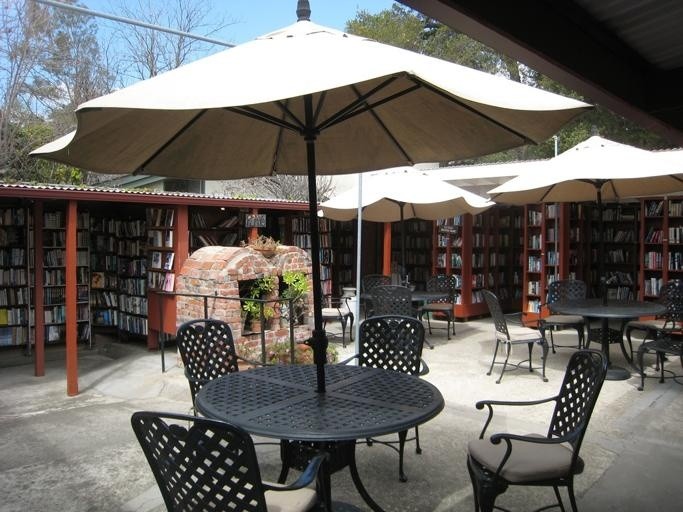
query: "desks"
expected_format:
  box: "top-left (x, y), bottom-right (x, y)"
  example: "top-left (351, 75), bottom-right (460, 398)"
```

top-left (195, 364), bottom-right (444, 512)
top-left (548, 297), bottom-right (667, 380)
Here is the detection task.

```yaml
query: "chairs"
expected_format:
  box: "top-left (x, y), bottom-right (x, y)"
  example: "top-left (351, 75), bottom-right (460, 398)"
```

top-left (465, 350), bottom-right (607, 512)
top-left (175, 319), bottom-right (282, 456)
top-left (335, 316), bottom-right (430, 483)
top-left (481, 288), bottom-right (549, 384)
top-left (131, 410), bottom-right (330, 512)
top-left (637, 282), bottom-right (683, 390)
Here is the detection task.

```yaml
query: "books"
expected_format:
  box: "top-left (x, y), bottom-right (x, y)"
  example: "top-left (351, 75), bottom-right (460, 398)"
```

top-left (0, 200), bottom-right (682, 347)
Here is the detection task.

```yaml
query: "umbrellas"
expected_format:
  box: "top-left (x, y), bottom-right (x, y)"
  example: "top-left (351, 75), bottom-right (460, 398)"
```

top-left (318, 166), bottom-right (497, 288)
top-left (28, 0), bottom-right (597, 394)
top-left (486, 135), bottom-right (683, 305)
